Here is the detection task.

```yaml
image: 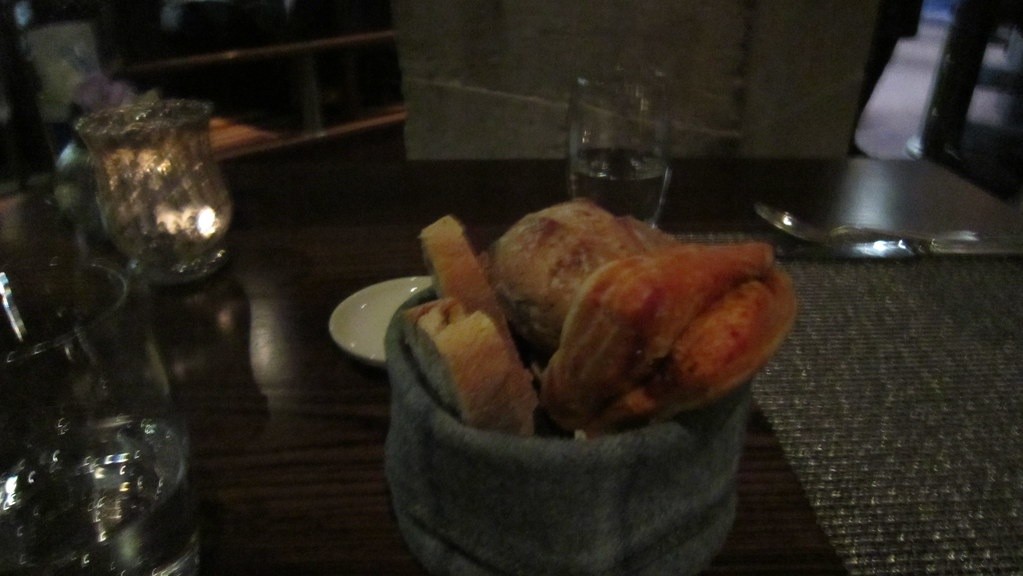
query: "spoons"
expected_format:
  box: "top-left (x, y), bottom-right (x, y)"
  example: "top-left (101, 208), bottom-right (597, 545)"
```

top-left (750, 202), bottom-right (1023, 265)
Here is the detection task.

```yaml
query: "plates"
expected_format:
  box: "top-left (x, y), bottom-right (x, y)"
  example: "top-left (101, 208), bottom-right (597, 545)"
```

top-left (328, 276), bottom-right (433, 367)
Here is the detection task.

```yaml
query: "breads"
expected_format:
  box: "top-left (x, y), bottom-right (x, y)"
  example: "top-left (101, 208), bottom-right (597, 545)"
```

top-left (475, 204), bottom-right (678, 347)
top-left (402, 299), bottom-right (523, 436)
top-left (537, 242), bottom-right (794, 434)
top-left (422, 213), bottom-right (520, 358)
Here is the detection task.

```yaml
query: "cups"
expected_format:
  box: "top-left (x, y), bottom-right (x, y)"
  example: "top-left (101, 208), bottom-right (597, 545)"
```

top-left (74, 95), bottom-right (232, 282)
top-left (0, 259), bottom-right (202, 575)
top-left (569, 71), bottom-right (671, 234)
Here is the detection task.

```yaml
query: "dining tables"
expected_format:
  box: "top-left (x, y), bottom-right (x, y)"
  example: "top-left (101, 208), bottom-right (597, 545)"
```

top-left (0, 156), bottom-right (1023, 576)
